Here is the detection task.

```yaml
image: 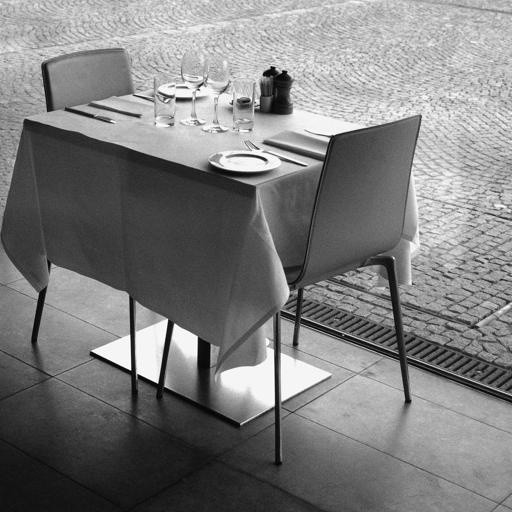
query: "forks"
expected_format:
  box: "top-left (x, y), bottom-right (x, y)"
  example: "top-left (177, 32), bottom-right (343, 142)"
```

top-left (244, 137), bottom-right (309, 167)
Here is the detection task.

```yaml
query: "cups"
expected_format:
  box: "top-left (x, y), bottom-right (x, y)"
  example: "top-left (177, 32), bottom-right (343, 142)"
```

top-left (260, 95), bottom-right (274, 111)
top-left (154, 74), bottom-right (176, 128)
top-left (230, 79), bottom-right (256, 131)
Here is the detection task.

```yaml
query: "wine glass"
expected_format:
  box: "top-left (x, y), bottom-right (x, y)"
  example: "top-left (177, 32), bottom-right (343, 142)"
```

top-left (180, 51), bottom-right (230, 135)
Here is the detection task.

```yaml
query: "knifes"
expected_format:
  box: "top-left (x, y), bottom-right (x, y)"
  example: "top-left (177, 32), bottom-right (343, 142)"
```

top-left (64, 106), bottom-right (117, 125)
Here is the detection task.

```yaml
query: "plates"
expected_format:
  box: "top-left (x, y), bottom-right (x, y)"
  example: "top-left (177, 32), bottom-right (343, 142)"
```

top-left (207, 151), bottom-right (281, 173)
top-left (158, 83), bottom-right (209, 99)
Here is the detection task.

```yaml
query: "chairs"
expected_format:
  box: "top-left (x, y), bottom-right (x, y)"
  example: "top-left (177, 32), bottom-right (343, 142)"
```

top-left (30, 48), bottom-right (140, 398)
top-left (155, 114), bottom-right (422, 466)
